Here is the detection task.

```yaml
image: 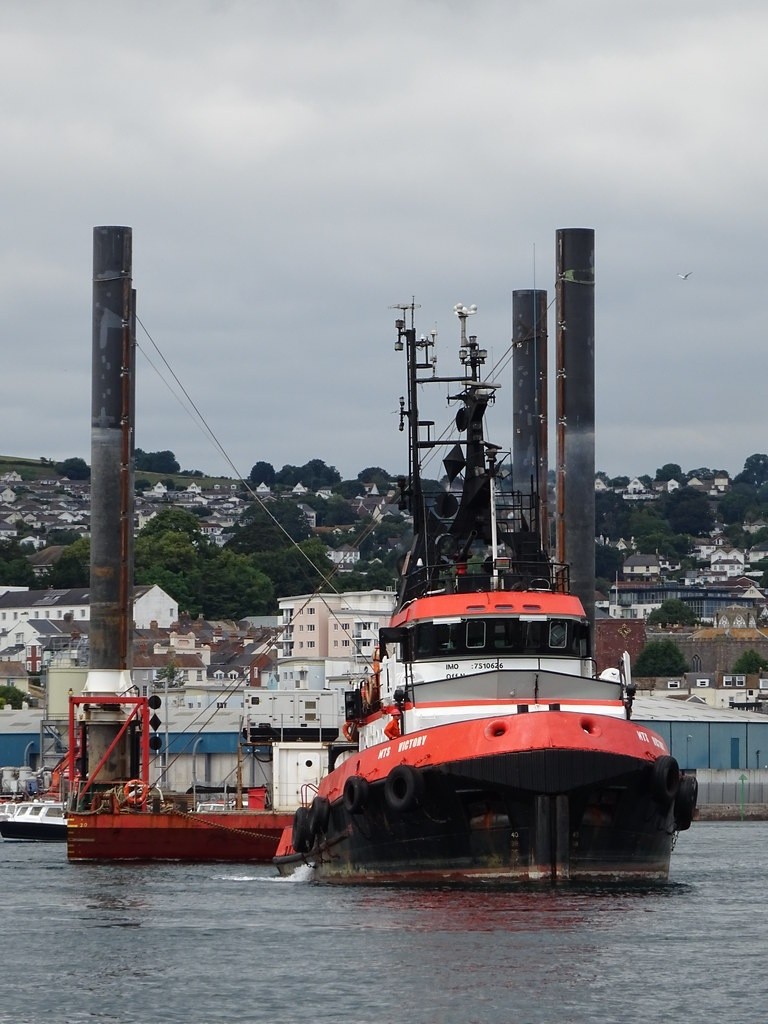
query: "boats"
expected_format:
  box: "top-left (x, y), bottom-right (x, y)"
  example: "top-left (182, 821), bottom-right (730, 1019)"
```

top-left (0, 801), bottom-right (68, 841)
top-left (272, 291), bottom-right (697, 884)
top-left (192, 802), bottom-right (247, 811)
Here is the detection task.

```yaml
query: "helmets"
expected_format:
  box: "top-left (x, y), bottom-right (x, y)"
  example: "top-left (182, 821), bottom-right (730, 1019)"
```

top-left (391, 709), bottom-right (401, 716)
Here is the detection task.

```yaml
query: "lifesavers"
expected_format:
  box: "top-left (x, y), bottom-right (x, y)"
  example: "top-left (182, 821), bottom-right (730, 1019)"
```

top-left (674, 775), bottom-right (698, 822)
top-left (365, 675), bottom-right (378, 706)
top-left (124, 779), bottom-right (149, 805)
top-left (652, 754), bottom-right (680, 802)
top-left (342, 775), bottom-right (370, 815)
top-left (342, 721), bottom-right (356, 742)
top-left (308, 797), bottom-right (331, 834)
top-left (291, 806), bottom-right (314, 854)
top-left (384, 764), bottom-right (422, 814)
